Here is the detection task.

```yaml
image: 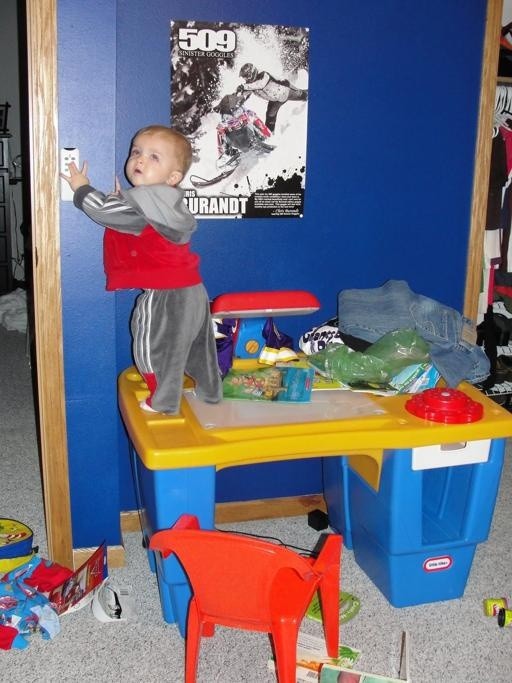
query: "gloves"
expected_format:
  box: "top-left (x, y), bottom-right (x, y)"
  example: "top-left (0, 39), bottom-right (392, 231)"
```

top-left (235, 85), bottom-right (244, 94)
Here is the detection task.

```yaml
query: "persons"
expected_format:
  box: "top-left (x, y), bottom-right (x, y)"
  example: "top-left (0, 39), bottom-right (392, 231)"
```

top-left (235, 64), bottom-right (308, 134)
top-left (59, 126), bottom-right (223, 414)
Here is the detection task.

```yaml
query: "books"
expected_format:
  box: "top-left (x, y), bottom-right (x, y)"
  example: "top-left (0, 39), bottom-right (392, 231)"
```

top-left (217, 365), bottom-right (315, 403)
top-left (267, 632), bottom-right (360, 682)
top-left (46, 539), bottom-right (131, 624)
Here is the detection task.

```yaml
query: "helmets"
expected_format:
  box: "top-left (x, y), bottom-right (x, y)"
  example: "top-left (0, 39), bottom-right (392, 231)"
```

top-left (239, 63), bottom-right (258, 82)
